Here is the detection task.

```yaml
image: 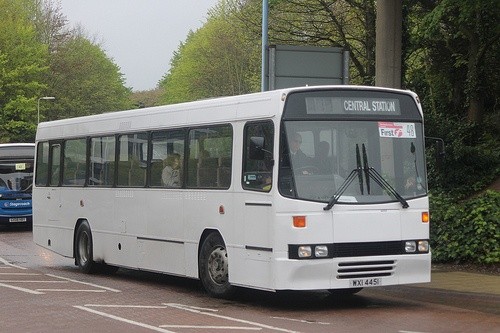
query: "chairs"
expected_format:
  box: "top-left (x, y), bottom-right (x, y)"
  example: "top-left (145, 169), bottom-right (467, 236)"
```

top-left (129, 159), bottom-right (144, 186)
top-left (215, 152), bottom-right (230, 187)
top-left (144, 157), bottom-right (163, 186)
top-left (195, 150), bottom-right (213, 188)
top-left (73, 162), bottom-right (86, 185)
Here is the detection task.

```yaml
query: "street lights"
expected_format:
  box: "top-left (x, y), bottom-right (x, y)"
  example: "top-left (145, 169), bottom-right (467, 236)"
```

top-left (37, 96), bottom-right (56, 124)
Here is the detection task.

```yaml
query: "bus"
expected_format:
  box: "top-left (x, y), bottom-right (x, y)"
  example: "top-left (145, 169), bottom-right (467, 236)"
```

top-left (0, 143), bottom-right (36, 230)
top-left (31, 85), bottom-right (447, 301)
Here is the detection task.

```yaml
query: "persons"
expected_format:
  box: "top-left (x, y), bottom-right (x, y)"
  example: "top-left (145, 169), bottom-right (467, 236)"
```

top-left (317, 140), bottom-right (346, 179)
top-left (282, 133), bottom-right (306, 168)
top-left (194, 149), bottom-right (211, 186)
top-left (160, 153), bottom-right (181, 185)
